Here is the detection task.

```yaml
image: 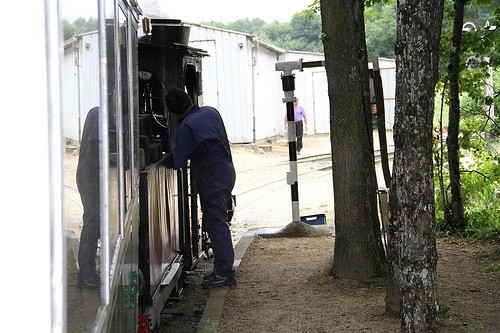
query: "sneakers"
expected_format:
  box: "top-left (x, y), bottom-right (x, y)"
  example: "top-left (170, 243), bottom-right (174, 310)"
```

top-left (77, 273), bottom-right (103, 288)
top-left (201, 273), bottom-right (236, 288)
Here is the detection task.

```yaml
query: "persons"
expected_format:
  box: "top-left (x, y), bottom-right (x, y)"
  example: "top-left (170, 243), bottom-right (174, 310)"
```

top-left (163, 87), bottom-right (236, 289)
top-left (285, 97), bottom-right (308, 154)
top-left (77, 107), bottom-right (100, 288)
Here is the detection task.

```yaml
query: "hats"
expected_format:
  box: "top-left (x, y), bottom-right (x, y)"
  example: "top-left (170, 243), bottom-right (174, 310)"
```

top-left (294, 97), bottom-right (299, 102)
